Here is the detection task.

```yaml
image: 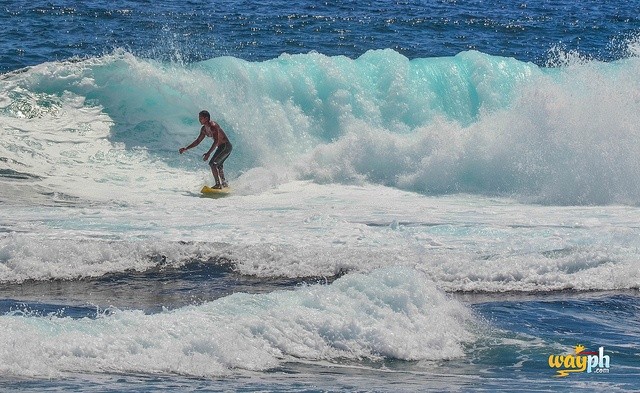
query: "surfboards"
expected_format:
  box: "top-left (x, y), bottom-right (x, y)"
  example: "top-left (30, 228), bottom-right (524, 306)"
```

top-left (201, 186), bottom-right (230, 194)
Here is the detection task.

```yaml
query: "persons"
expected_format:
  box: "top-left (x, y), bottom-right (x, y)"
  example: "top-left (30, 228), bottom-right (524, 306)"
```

top-left (179, 109), bottom-right (233, 188)
